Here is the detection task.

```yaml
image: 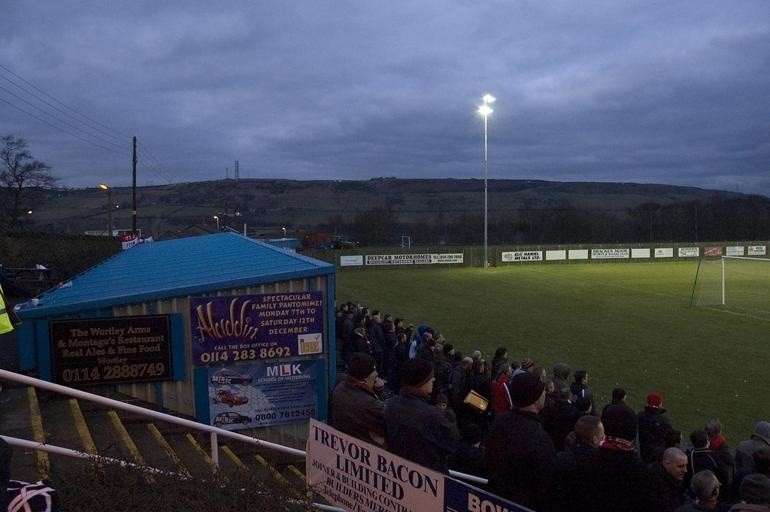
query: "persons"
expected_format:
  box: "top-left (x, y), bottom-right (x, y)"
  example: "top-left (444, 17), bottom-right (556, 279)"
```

top-left (328, 301), bottom-right (769, 511)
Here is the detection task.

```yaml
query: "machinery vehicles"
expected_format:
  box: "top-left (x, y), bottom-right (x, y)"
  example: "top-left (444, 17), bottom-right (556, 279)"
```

top-left (319, 235), bottom-right (360, 251)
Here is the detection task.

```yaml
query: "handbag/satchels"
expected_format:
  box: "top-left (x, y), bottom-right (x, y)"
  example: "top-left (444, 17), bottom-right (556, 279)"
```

top-left (462, 388), bottom-right (489, 415)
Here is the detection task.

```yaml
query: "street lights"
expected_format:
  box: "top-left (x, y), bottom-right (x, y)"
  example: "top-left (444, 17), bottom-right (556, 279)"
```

top-left (98, 182), bottom-right (113, 238)
top-left (214, 216), bottom-right (219, 231)
top-left (282, 227), bottom-right (287, 236)
top-left (476, 92), bottom-right (497, 267)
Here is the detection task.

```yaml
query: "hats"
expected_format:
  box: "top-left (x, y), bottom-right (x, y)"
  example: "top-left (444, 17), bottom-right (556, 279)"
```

top-left (400, 357), bottom-right (435, 387)
top-left (521, 358), bottom-right (533, 370)
top-left (511, 372), bottom-right (543, 407)
top-left (347, 351), bottom-right (375, 378)
top-left (436, 393), bottom-right (449, 403)
top-left (647, 394), bottom-right (662, 406)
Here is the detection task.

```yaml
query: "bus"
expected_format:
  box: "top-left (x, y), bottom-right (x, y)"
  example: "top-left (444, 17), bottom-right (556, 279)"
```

top-left (84, 228), bottom-right (141, 238)
